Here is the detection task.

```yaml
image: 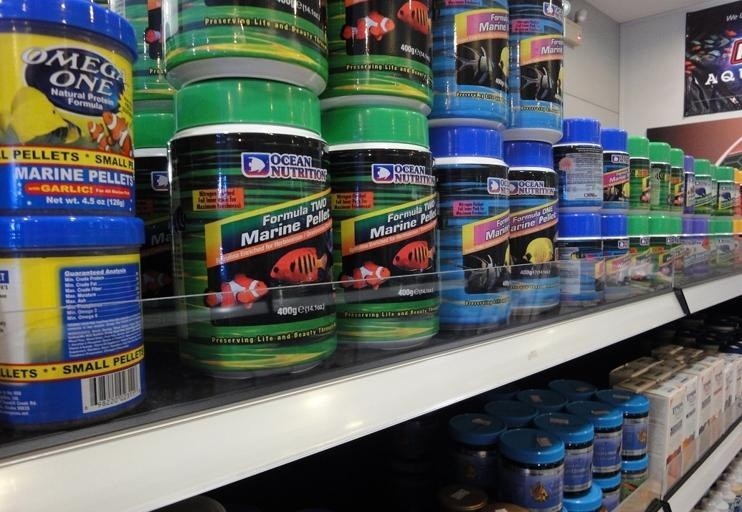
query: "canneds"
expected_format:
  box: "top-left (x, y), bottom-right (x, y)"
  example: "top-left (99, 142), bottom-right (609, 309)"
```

top-left (1, 0), bottom-right (741, 512)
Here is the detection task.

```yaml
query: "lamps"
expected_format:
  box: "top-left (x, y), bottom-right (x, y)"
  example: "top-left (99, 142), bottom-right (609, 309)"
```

top-left (562, 0), bottom-right (588, 47)
